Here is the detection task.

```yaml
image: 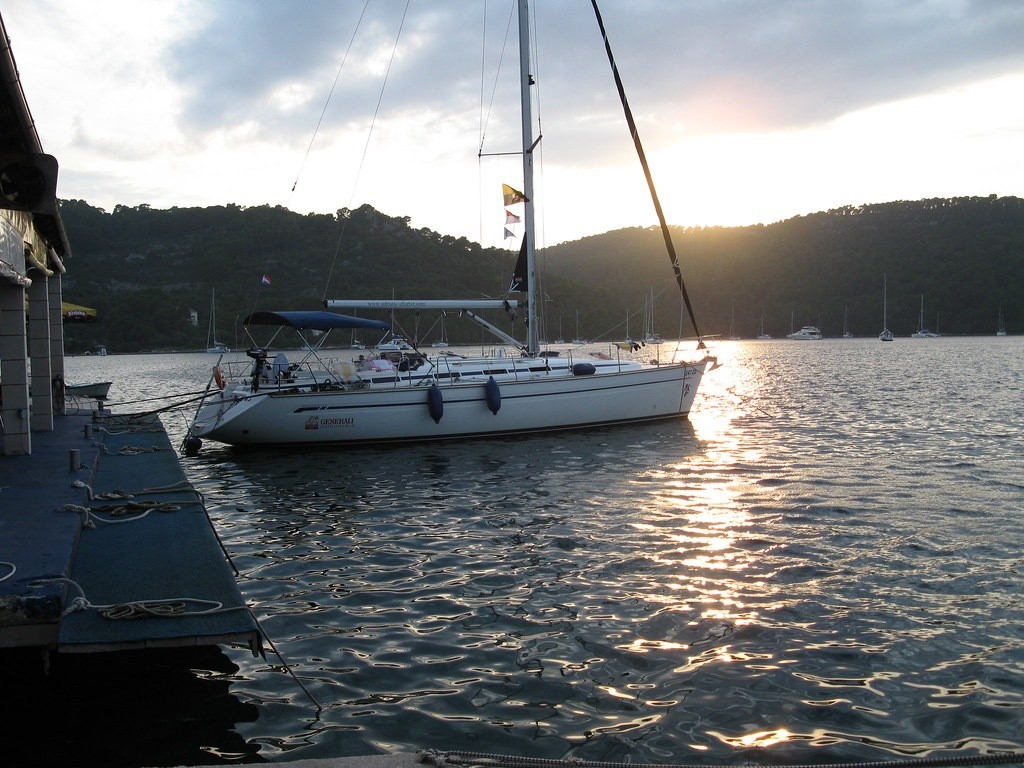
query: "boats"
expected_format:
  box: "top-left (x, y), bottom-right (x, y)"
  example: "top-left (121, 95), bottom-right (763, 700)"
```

top-left (66, 382), bottom-right (113, 400)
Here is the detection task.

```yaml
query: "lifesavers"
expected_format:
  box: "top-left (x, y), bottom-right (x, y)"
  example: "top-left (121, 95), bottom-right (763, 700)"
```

top-left (212, 365), bottom-right (227, 390)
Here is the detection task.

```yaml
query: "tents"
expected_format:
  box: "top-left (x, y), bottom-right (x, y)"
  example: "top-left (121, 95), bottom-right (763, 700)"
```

top-left (23, 294), bottom-right (97, 318)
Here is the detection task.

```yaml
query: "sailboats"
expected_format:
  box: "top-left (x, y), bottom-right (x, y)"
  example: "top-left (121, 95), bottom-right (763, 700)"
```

top-left (912, 294), bottom-right (937, 337)
top-left (728, 306), bottom-right (740, 340)
top-left (879, 273), bottom-right (895, 341)
top-left (758, 313), bottom-right (771, 340)
top-left (181, 1), bottom-right (722, 456)
top-left (996, 309), bottom-right (1008, 336)
top-left (784, 312), bottom-right (824, 341)
top-left (842, 304), bottom-right (854, 338)
